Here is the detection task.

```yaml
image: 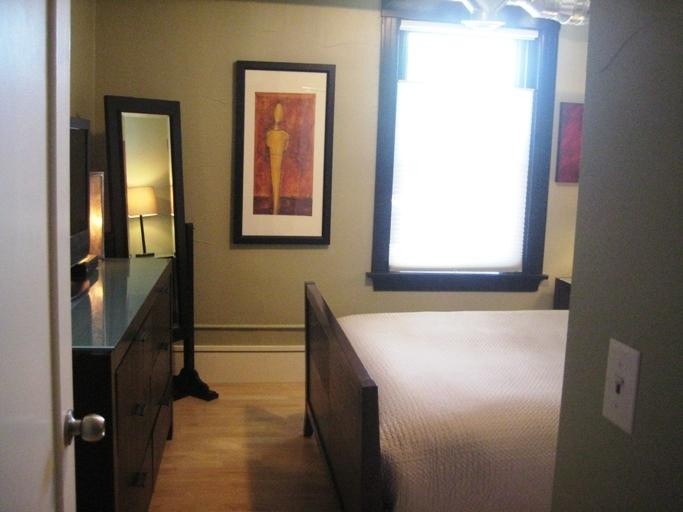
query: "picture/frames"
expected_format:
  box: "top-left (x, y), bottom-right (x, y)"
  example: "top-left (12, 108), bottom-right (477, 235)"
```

top-left (233, 60), bottom-right (336, 244)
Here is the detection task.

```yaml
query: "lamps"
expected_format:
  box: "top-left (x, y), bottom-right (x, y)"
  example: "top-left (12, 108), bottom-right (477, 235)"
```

top-left (126, 185), bottom-right (159, 257)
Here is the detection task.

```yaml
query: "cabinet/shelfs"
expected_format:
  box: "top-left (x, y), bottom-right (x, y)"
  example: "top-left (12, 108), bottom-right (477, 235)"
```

top-left (71, 256), bottom-right (173, 512)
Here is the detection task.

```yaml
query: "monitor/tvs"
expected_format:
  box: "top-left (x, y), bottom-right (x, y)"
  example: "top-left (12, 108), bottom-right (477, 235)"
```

top-left (70, 117), bottom-right (91, 304)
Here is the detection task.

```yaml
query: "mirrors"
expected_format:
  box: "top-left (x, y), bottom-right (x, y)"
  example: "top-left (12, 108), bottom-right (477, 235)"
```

top-left (104, 95), bottom-right (218, 402)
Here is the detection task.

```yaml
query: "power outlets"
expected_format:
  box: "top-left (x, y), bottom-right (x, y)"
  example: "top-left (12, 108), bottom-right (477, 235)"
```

top-left (601, 337), bottom-right (641, 435)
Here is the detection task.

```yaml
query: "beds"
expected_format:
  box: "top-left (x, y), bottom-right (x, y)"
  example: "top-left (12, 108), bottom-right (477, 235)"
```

top-left (304, 282), bottom-right (570, 512)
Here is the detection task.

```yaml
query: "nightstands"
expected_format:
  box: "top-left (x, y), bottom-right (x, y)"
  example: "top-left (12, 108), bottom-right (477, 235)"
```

top-left (554, 277), bottom-right (571, 310)
